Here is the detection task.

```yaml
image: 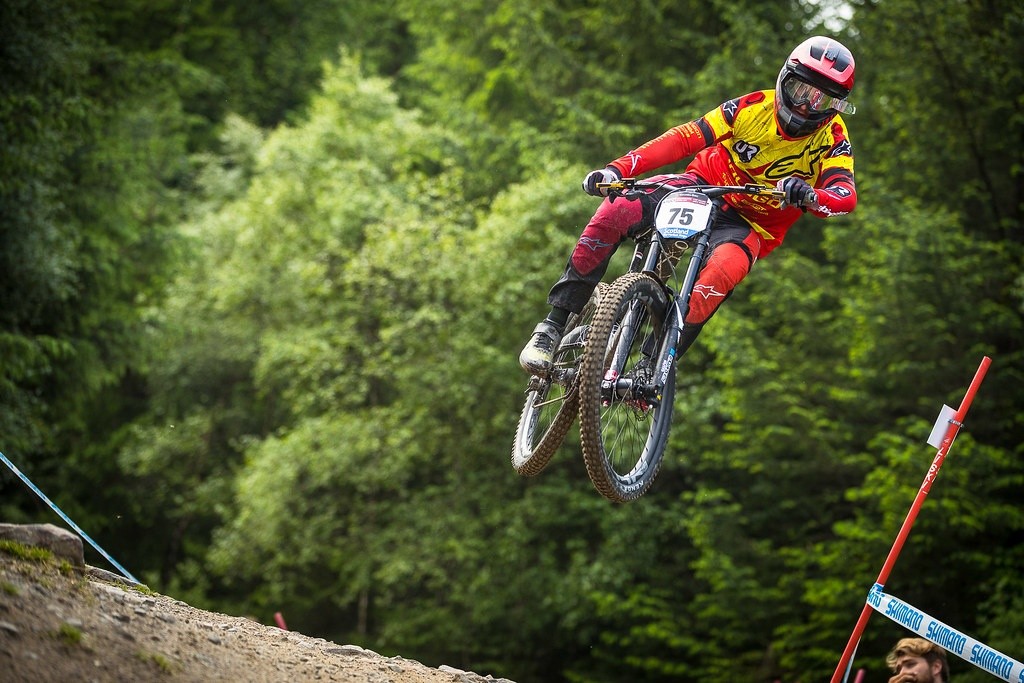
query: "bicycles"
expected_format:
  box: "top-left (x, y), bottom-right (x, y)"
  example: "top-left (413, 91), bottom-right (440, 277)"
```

top-left (510, 181), bottom-right (817, 504)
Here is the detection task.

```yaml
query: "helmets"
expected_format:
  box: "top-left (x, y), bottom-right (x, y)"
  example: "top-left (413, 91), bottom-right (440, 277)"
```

top-left (775, 36), bottom-right (856, 136)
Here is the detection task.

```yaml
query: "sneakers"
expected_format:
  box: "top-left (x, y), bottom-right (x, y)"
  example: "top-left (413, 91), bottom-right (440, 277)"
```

top-left (519, 319), bottom-right (561, 377)
top-left (625, 356), bottom-right (656, 384)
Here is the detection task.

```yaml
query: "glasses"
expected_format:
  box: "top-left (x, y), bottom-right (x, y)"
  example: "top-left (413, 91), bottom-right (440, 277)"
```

top-left (781, 70), bottom-right (841, 115)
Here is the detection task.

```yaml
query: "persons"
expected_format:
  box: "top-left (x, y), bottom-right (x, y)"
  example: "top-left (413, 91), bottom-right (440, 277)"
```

top-left (887, 638), bottom-right (950, 683)
top-left (519, 36), bottom-right (857, 408)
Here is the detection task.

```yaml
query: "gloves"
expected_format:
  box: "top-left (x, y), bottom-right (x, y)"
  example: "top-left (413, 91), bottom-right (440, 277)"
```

top-left (777, 177), bottom-right (814, 214)
top-left (583, 168), bottom-right (621, 199)
